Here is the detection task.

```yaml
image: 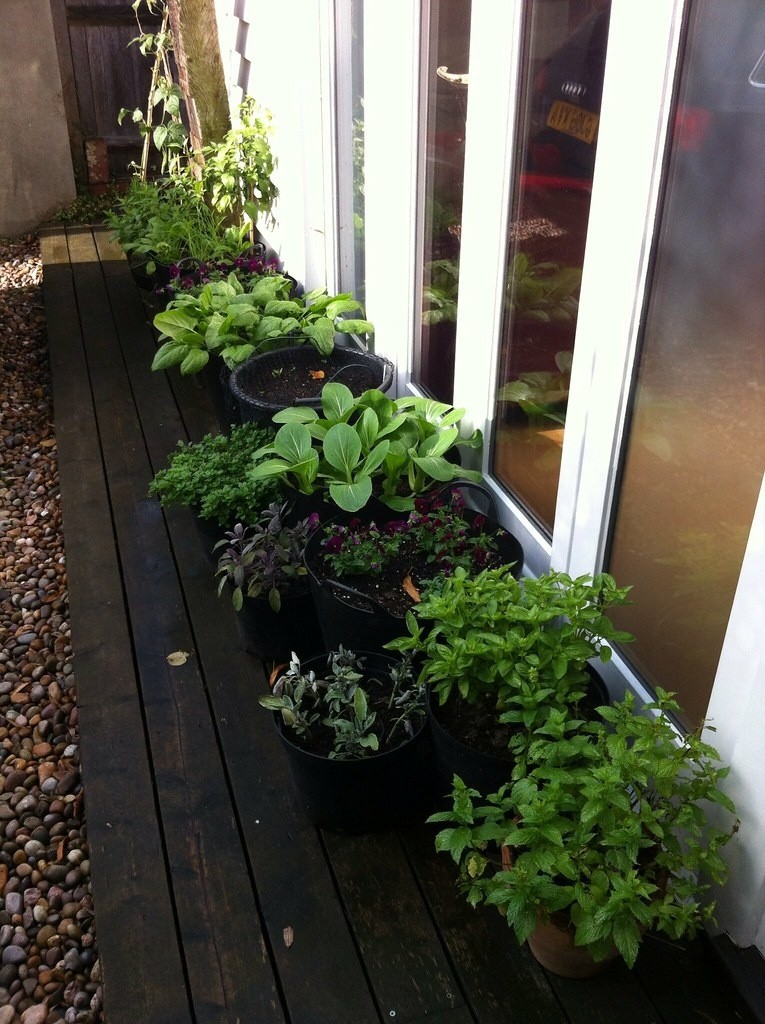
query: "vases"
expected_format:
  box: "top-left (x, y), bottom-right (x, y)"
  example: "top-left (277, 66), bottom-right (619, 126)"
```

top-left (302, 516), bottom-right (525, 659)
top-left (177, 258), bottom-right (299, 301)
top-left (231, 347), bottom-right (395, 424)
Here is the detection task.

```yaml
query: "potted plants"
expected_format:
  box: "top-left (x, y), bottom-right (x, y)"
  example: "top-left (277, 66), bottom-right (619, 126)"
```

top-left (104, 93), bottom-right (375, 434)
top-left (142, 379), bottom-right (742, 980)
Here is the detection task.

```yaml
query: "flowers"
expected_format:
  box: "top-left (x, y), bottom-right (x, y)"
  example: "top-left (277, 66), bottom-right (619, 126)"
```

top-left (154, 255), bottom-right (280, 295)
top-left (308, 486), bottom-right (509, 575)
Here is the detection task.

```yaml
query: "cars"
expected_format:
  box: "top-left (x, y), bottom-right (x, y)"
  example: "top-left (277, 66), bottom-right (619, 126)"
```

top-left (526, 10), bottom-right (748, 192)
